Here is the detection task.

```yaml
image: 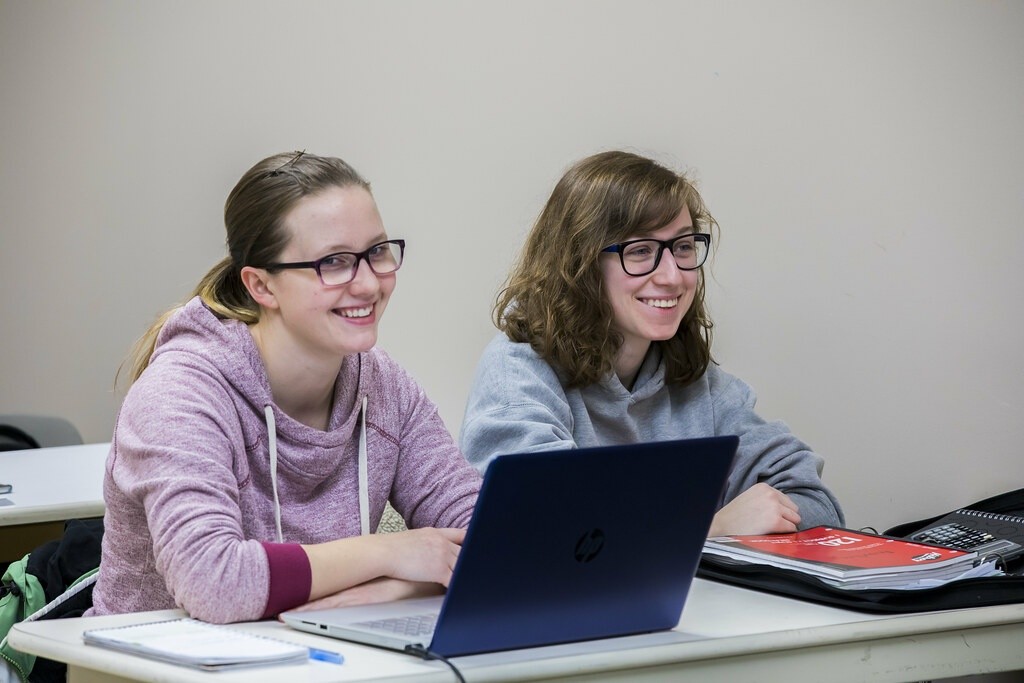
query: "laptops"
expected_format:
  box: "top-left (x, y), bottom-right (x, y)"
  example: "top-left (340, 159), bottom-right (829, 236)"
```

top-left (278, 434), bottom-right (740, 659)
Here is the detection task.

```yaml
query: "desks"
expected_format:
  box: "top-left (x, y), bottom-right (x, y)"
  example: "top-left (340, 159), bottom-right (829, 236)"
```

top-left (0, 441), bottom-right (1024, 683)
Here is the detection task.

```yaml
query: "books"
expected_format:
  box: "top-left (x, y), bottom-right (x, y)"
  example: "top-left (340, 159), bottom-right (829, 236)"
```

top-left (899, 508), bottom-right (1024, 565)
top-left (84, 618), bottom-right (309, 671)
top-left (702, 525), bottom-right (979, 591)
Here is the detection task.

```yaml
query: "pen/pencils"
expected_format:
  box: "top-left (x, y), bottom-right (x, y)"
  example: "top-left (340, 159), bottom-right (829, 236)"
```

top-left (309, 648), bottom-right (344, 663)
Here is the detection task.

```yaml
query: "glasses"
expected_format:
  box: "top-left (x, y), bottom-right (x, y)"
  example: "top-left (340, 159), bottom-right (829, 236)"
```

top-left (600, 232), bottom-right (711, 274)
top-left (265, 238), bottom-right (405, 286)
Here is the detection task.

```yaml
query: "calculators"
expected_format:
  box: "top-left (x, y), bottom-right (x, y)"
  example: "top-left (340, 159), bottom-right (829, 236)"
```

top-left (911, 523), bottom-right (1024, 566)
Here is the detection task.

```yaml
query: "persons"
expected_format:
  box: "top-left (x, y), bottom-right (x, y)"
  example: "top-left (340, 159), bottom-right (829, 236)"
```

top-left (83, 149), bottom-right (484, 625)
top-left (460, 151), bottom-right (845, 536)
